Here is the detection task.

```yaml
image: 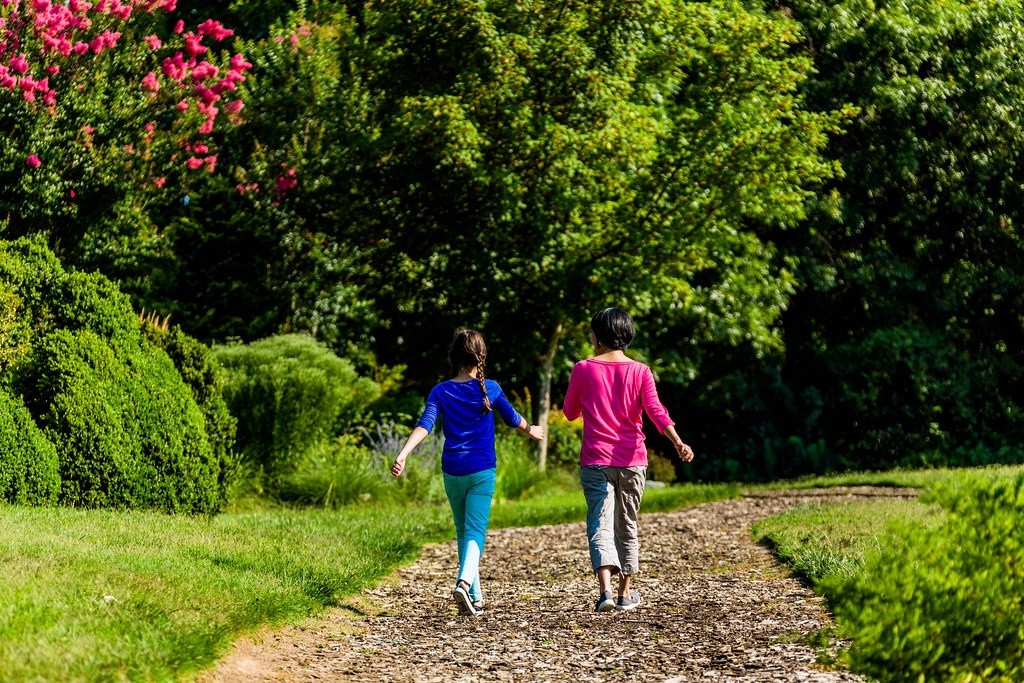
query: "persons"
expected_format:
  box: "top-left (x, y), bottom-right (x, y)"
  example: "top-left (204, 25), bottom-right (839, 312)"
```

top-left (391, 326), bottom-right (545, 618)
top-left (562, 307), bottom-right (693, 611)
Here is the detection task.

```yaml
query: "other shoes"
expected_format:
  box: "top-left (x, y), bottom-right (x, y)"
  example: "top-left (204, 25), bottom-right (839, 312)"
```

top-left (595, 592), bottom-right (615, 612)
top-left (472, 600), bottom-right (486, 613)
top-left (452, 584), bottom-right (476, 618)
top-left (617, 591), bottom-right (642, 612)
